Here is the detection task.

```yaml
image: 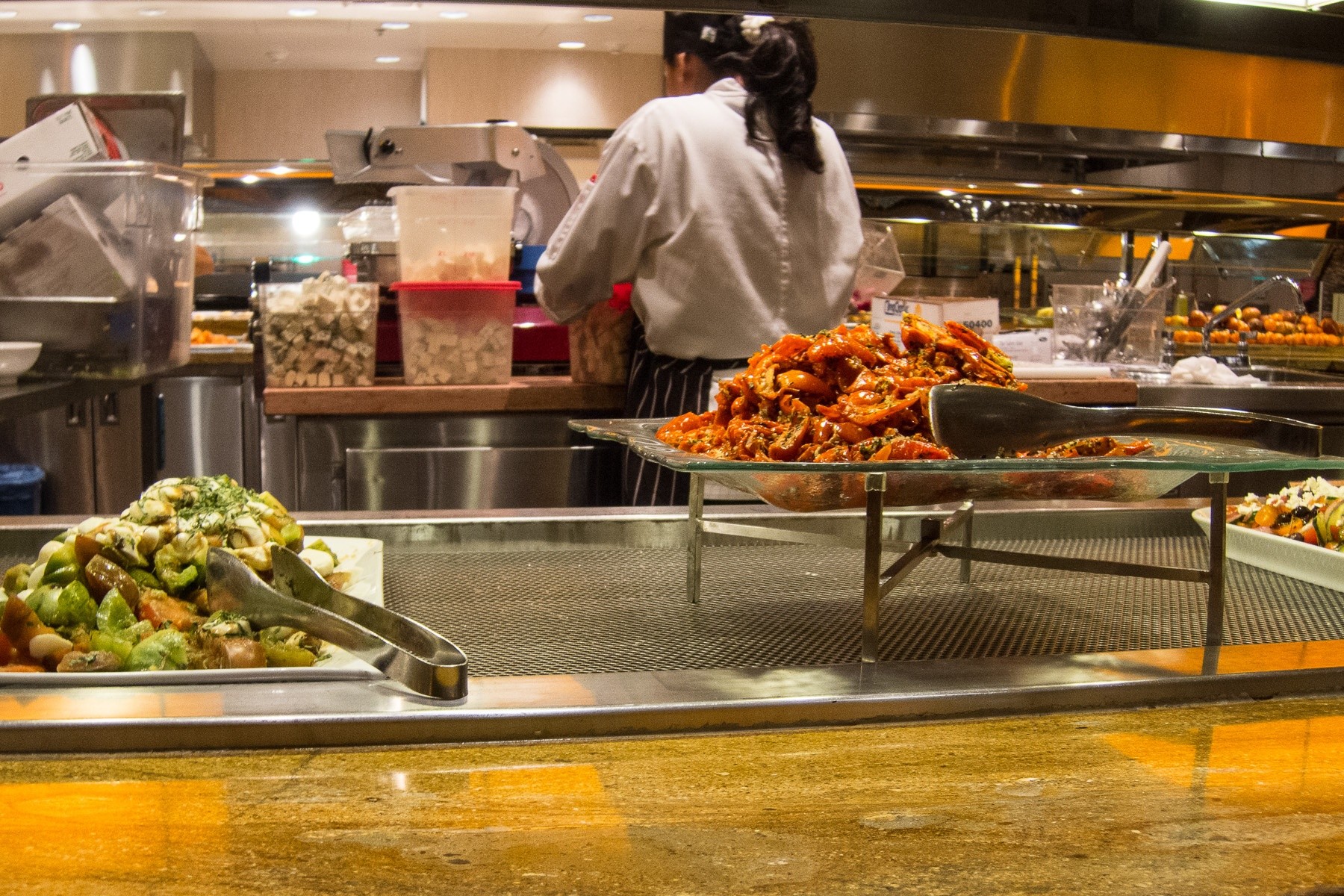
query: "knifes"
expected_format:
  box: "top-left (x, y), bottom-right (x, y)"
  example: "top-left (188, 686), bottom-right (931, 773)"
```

top-left (1107, 241), bottom-right (1171, 339)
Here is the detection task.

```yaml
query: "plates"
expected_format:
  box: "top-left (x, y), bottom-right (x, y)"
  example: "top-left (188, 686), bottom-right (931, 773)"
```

top-left (0, 535), bottom-right (387, 684)
top-left (190, 344), bottom-right (253, 351)
top-left (1192, 505), bottom-right (1344, 593)
top-left (567, 416), bottom-right (1344, 514)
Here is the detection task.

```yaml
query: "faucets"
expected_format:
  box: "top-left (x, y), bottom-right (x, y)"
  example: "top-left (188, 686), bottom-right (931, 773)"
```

top-left (1194, 272), bottom-right (1307, 365)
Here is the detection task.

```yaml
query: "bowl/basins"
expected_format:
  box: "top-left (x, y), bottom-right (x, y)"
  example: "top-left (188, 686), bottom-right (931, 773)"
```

top-left (0, 341), bottom-right (43, 385)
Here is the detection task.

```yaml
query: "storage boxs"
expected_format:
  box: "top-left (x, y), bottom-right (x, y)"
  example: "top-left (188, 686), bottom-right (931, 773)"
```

top-left (258, 282), bottom-right (379, 388)
top-left (0, 193), bottom-right (137, 296)
top-left (0, 163), bottom-right (215, 389)
top-left (0, 101), bottom-right (131, 232)
top-left (871, 297), bottom-right (1001, 352)
top-left (569, 284), bottom-right (637, 386)
top-left (1052, 284), bottom-right (1168, 364)
top-left (386, 186), bottom-right (523, 386)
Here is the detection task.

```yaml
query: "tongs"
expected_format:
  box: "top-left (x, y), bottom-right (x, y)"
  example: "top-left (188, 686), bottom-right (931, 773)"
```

top-left (929, 385), bottom-right (1321, 458)
top-left (205, 545), bottom-right (468, 700)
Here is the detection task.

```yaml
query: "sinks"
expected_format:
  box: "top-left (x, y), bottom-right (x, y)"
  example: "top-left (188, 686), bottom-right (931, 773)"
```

top-left (1226, 364), bottom-right (1344, 387)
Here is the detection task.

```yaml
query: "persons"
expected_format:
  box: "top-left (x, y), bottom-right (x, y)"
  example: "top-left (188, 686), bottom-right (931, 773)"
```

top-left (533, 9), bottom-right (866, 507)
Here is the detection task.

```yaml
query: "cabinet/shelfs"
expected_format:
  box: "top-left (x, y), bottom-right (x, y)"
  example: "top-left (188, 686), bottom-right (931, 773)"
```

top-left (1, 379), bottom-right (153, 516)
top-left (146, 375), bottom-right (243, 486)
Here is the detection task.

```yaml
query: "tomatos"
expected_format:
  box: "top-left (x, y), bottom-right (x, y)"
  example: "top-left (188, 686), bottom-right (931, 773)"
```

top-left (0, 491), bottom-right (346, 673)
top-left (1224, 497), bottom-right (1344, 553)
top-left (658, 318), bottom-right (1152, 511)
top-left (1162, 304), bottom-right (1344, 347)
